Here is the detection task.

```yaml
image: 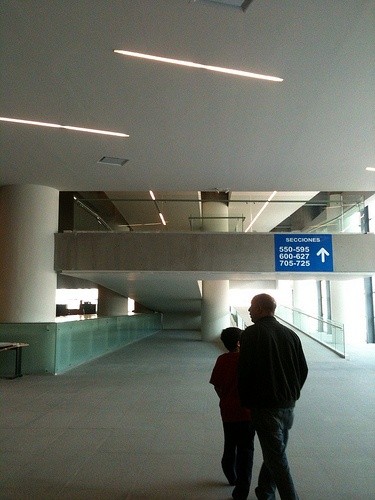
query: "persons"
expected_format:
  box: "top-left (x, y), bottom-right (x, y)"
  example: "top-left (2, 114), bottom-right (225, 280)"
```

top-left (209, 327), bottom-right (257, 499)
top-left (237, 293), bottom-right (310, 500)
